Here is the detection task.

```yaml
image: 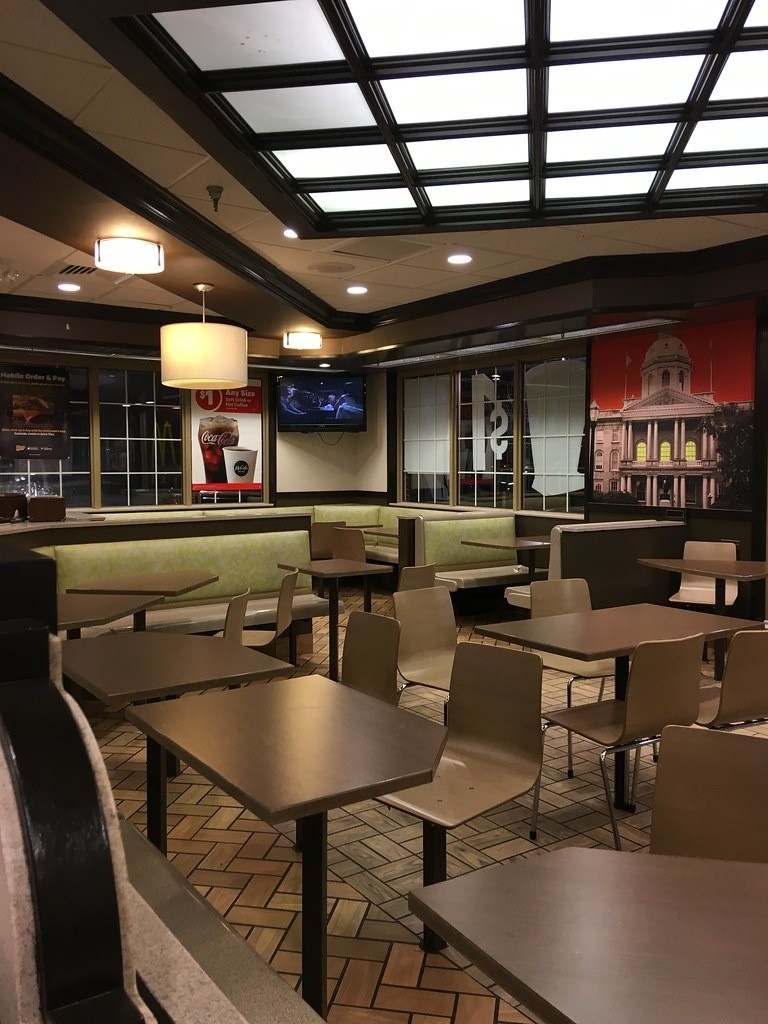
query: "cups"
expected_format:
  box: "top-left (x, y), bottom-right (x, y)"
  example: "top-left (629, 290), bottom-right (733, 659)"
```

top-left (198, 419), bottom-right (239, 484)
top-left (222, 447), bottom-right (258, 483)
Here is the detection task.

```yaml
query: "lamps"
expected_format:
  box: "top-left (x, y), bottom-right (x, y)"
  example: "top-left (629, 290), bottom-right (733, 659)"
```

top-left (160, 284), bottom-right (248, 389)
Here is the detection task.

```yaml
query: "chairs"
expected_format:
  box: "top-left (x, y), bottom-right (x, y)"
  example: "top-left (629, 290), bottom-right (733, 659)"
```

top-left (213, 521), bottom-right (768, 953)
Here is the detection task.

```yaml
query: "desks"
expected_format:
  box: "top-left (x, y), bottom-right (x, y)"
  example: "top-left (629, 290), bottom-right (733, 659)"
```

top-left (57, 594), bottom-right (165, 708)
top-left (475, 603), bottom-right (765, 810)
top-left (462, 534), bottom-right (551, 620)
top-left (406, 846), bottom-right (768, 1024)
top-left (343, 524), bottom-right (384, 528)
top-left (363, 527), bottom-right (398, 538)
top-left (61, 631), bottom-right (294, 862)
top-left (637, 558), bottom-right (768, 681)
top-left (124, 675), bottom-right (451, 1019)
top-left (67, 570), bottom-right (219, 707)
top-left (275, 559), bottom-right (393, 682)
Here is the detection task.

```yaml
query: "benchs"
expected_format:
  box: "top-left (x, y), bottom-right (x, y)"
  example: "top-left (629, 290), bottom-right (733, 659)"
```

top-left (0, 505), bottom-right (688, 1024)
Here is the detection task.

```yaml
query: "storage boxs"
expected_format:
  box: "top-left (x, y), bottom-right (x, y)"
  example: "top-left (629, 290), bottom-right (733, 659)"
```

top-left (0, 493), bottom-right (66, 521)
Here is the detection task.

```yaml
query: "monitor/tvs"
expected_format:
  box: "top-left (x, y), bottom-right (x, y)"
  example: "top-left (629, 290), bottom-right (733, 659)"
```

top-left (277, 374), bottom-right (367, 432)
